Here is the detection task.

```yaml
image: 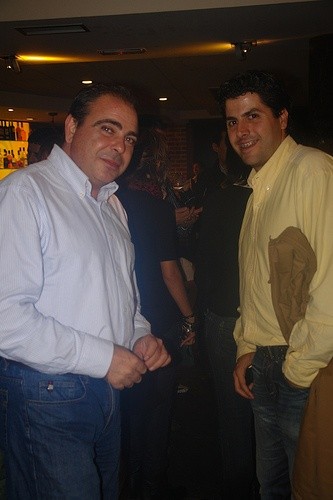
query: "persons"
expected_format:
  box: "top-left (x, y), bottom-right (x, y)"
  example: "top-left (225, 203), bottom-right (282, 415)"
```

top-left (191, 142), bottom-right (262, 500)
top-left (0, 83), bottom-right (171, 500)
top-left (112, 146), bottom-right (196, 500)
top-left (291, 358), bottom-right (333, 500)
top-left (193, 127), bottom-right (237, 209)
top-left (126, 129), bottom-right (200, 228)
top-left (217, 78), bottom-right (333, 500)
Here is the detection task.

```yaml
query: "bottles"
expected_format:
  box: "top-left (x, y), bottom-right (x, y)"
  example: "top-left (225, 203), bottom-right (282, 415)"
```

top-left (0, 120), bottom-right (23, 141)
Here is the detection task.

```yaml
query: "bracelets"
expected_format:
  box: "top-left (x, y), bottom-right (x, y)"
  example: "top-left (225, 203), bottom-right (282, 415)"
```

top-left (185, 313), bottom-right (195, 319)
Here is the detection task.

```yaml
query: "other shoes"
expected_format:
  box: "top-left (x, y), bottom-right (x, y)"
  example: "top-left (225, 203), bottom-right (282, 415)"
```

top-left (176, 384), bottom-right (189, 393)
